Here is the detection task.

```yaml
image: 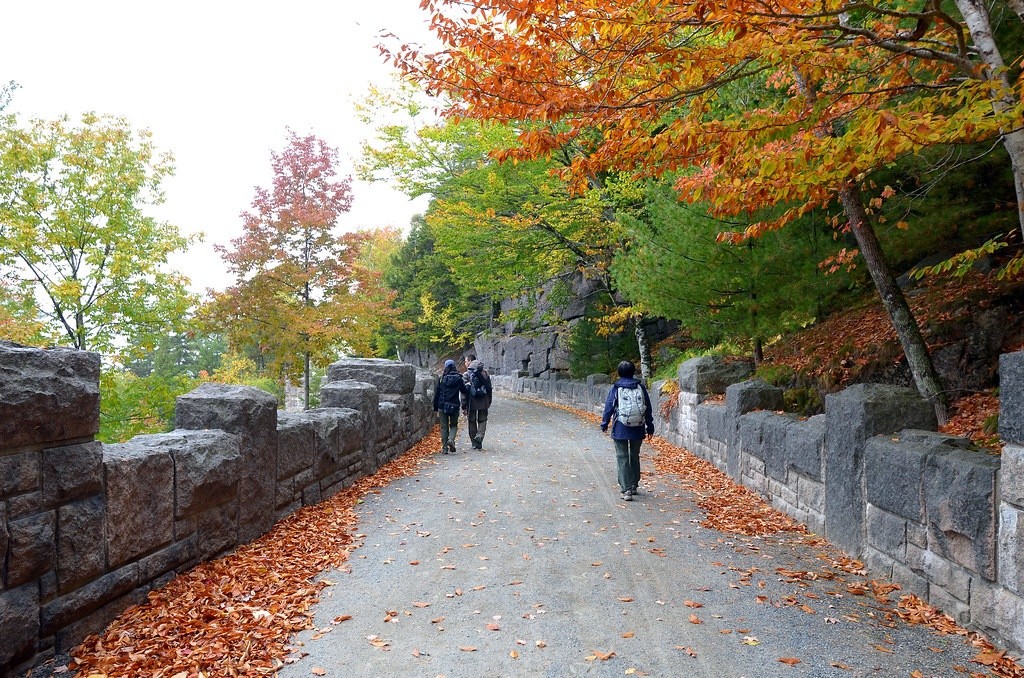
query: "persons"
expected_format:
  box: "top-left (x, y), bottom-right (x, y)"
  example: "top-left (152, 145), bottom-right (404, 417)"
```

top-left (460, 354), bottom-right (492, 450)
top-left (599, 360), bottom-right (654, 501)
top-left (433, 360), bottom-right (471, 455)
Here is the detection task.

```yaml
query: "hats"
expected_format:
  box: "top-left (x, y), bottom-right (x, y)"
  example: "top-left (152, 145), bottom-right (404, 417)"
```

top-left (445, 360), bottom-right (455, 367)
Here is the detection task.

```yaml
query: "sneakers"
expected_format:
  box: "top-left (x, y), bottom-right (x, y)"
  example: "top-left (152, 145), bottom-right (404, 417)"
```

top-left (620, 490), bottom-right (631, 500)
top-left (631, 489), bottom-right (637, 495)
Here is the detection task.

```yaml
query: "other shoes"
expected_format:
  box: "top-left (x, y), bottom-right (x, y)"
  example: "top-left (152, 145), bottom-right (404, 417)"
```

top-left (472, 444), bottom-right (476, 449)
top-left (443, 448), bottom-right (448, 454)
top-left (474, 437), bottom-right (482, 449)
top-left (447, 440), bottom-right (456, 452)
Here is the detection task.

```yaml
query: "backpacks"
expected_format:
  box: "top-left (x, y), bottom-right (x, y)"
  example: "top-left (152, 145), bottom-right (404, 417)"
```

top-left (615, 385), bottom-right (647, 427)
top-left (468, 370), bottom-right (487, 399)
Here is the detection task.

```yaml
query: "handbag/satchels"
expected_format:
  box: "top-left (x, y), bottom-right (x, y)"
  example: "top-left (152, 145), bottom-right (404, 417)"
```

top-left (443, 402), bottom-right (459, 414)
